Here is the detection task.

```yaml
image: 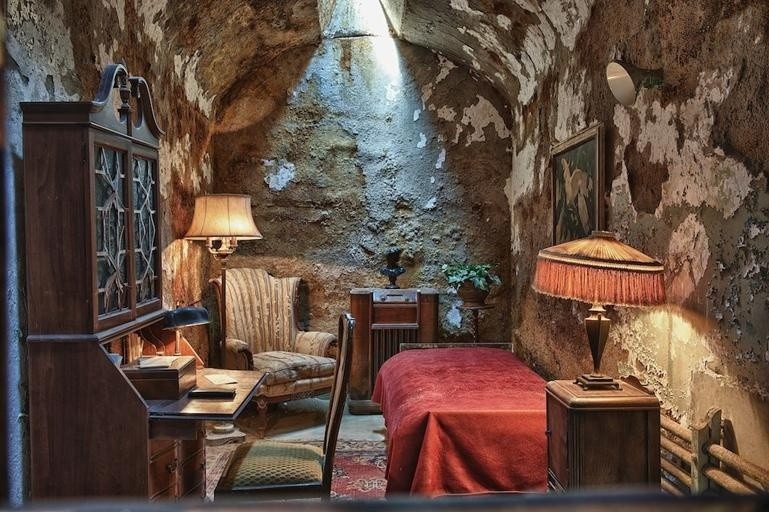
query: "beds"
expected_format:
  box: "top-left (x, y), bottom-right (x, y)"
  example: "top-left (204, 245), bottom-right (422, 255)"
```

top-left (371, 342), bottom-right (548, 500)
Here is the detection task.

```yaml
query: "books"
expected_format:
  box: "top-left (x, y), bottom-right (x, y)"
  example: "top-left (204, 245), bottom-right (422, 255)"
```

top-left (188, 387), bottom-right (237, 398)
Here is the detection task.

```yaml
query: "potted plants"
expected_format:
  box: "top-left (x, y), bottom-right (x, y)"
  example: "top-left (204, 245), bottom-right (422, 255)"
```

top-left (442, 263), bottom-right (502, 307)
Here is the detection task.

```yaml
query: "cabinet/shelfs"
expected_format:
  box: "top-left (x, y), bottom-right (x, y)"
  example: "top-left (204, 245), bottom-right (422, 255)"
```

top-left (19, 65), bottom-right (268, 504)
top-left (545, 379), bottom-right (661, 496)
top-left (347, 287), bottom-right (440, 415)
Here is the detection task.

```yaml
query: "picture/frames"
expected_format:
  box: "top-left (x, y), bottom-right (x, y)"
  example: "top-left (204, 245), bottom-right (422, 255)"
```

top-left (549, 121), bottom-right (606, 247)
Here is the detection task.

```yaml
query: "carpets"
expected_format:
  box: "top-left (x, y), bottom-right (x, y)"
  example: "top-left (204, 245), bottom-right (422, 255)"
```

top-left (207, 439), bottom-right (387, 502)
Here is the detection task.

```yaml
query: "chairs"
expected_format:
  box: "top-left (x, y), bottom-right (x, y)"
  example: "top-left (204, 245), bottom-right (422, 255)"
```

top-left (208, 267), bottom-right (337, 438)
top-left (214, 313), bottom-right (356, 503)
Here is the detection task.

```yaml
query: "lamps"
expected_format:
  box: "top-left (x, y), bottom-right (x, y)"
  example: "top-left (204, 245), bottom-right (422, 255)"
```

top-left (606, 59), bottom-right (664, 107)
top-left (162, 306), bottom-right (211, 356)
top-left (531, 230), bottom-right (666, 391)
top-left (183, 193), bottom-right (263, 446)
